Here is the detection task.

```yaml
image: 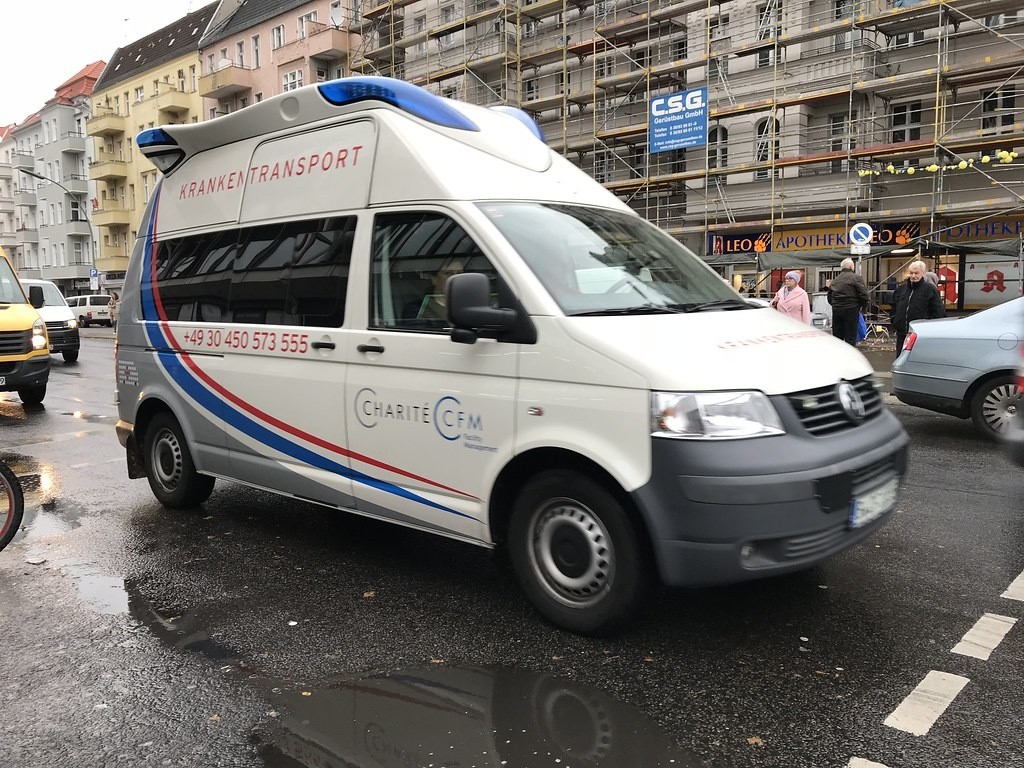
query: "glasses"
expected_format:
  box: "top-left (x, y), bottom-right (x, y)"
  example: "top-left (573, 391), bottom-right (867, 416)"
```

top-left (785, 277), bottom-right (794, 282)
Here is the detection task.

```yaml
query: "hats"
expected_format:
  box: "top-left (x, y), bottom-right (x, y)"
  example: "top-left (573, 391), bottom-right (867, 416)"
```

top-left (785, 270), bottom-right (801, 283)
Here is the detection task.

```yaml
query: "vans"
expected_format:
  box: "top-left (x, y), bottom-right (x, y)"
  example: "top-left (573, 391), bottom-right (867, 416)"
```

top-left (66, 295), bottom-right (114, 328)
top-left (115, 75), bottom-right (917, 631)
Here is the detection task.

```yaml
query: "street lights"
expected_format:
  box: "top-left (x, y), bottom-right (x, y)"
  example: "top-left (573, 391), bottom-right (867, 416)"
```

top-left (20, 168), bottom-right (98, 295)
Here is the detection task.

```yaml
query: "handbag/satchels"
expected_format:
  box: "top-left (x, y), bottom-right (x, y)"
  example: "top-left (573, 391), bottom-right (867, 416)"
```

top-left (855, 310), bottom-right (867, 342)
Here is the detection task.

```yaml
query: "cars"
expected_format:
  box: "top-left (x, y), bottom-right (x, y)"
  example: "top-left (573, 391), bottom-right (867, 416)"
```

top-left (0, 247), bottom-right (50, 406)
top-left (1, 278), bottom-right (81, 363)
top-left (887, 292), bottom-right (1024, 444)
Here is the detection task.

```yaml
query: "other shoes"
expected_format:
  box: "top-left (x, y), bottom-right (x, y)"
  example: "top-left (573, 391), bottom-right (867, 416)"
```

top-left (113, 332), bottom-right (116, 334)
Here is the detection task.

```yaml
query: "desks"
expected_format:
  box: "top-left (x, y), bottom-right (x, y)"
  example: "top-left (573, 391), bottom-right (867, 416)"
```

top-left (863, 321), bottom-right (891, 341)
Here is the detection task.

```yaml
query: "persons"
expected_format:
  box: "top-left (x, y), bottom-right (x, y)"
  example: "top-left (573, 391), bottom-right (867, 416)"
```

top-left (402, 249), bottom-right (468, 328)
top-left (922, 271), bottom-right (939, 289)
top-left (827, 257), bottom-right (868, 347)
top-left (889, 260), bottom-right (946, 361)
top-left (819, 277), bottom-right (831, 292)
top-left (108, 291), bottom-right (121, 334)
top-left (770, 270), bottom-right (811, 326)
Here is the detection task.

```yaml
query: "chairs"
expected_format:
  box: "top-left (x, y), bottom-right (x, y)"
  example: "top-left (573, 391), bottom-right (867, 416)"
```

top-left (392, 278), bottom-right (425, 328)
